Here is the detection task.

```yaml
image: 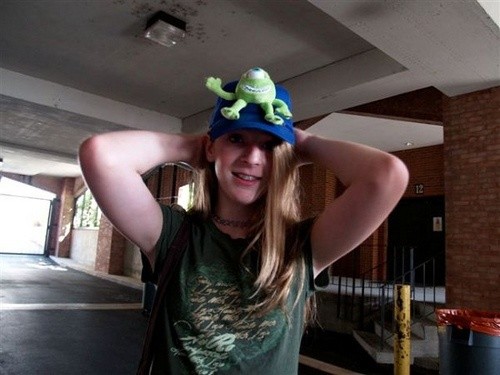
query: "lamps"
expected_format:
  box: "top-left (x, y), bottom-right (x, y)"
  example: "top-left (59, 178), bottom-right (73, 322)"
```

top-left (142, 9), bottom-right (189, 52)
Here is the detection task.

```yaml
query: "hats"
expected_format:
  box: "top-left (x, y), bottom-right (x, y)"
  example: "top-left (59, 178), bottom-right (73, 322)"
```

top-left (209, 82), bottom-right (295, 144)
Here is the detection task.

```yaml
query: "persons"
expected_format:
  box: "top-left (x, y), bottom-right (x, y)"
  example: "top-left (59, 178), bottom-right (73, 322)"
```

top-left (78, 69), bottom-right (411, 375)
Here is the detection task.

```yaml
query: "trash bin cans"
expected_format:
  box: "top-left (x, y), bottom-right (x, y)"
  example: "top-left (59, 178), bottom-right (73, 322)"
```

top-left (435, 306), bottom-right (500, 375)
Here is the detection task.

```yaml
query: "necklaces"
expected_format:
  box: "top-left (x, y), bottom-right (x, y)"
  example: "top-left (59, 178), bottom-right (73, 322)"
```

top-left (212, 211), bottom-right (258, 231)
